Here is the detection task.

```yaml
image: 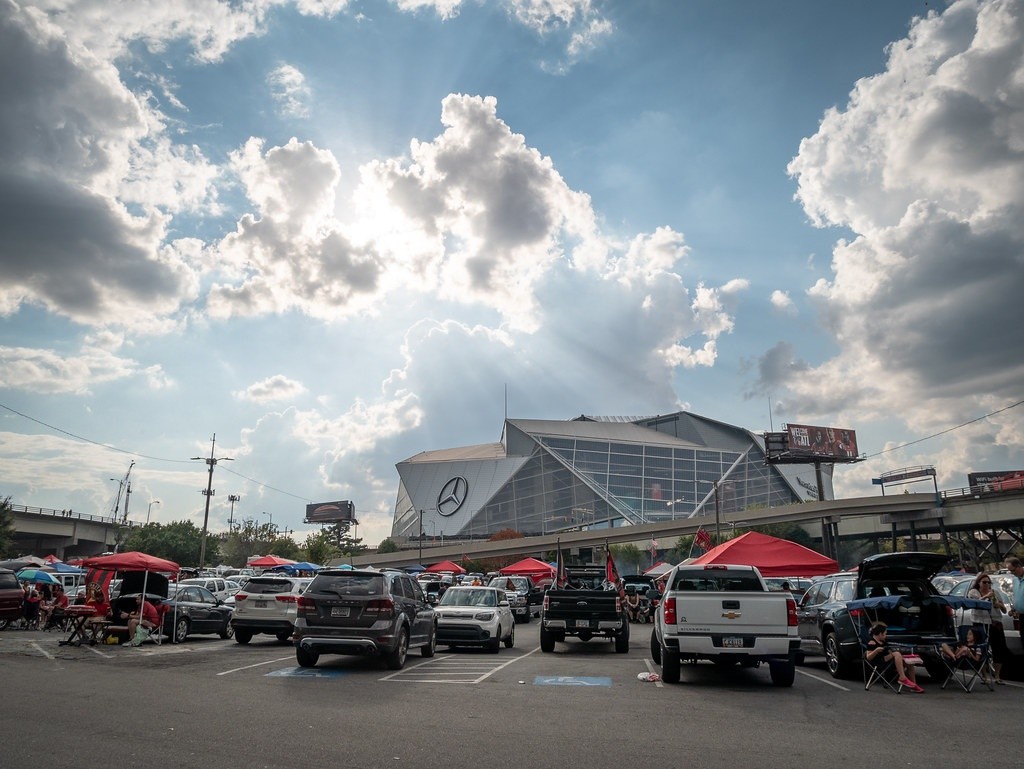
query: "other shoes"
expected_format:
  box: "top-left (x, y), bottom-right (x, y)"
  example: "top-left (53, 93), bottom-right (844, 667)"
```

top-left (897, 676), bottom-right (917, 688)
top-left (910, 684), bottom-right (925, 693)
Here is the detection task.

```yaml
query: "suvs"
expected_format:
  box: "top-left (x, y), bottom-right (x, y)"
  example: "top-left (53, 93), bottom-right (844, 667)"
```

top-left (292, 567), bottom-right (440, 671)
top-left (230, 575), bottom-right (313, 645)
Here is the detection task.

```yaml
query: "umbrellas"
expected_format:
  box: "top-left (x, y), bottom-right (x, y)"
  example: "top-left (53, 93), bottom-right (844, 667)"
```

top-left (16, 567), bottom-right (63, 585)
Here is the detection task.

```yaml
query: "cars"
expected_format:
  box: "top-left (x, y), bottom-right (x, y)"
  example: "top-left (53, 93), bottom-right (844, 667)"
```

top-left (148, 583), bottom-right (233, 644)
top-left (413, 569), bottom-right (545, 623)
top-left (433, 585), bottom-right (515, 654)
top-left (761, 565), bottom-right (1024, 681)
top-left (796, 551), bottom-right (961, 680)
top-left (621, 581), bottom-right (663, 625)
top-left (0, 563), bottom-right (316, 632)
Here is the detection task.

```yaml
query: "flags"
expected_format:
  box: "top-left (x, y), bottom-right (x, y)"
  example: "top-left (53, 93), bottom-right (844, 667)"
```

top-left (694, 528), bottom-right (714, 552)
top-left (607, 550), bottom-right (621, 588)
top-left (556, 544), bottom-right (568, 590)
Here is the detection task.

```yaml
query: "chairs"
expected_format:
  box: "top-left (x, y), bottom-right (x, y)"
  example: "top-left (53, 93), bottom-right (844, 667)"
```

top-left (24, 600), bottom-right (40, 630)
top-left (859, 624), bottom-right (904, 694)
top-left (935, 624), bottom-right (992, 693)
top-left (136, 604), bottom-right (170, 645)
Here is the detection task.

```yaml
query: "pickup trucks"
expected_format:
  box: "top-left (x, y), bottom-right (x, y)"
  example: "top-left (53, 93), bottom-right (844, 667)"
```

top-left (540, 565), bottom-right (630, 654)
top-left (645, 563), bottom-right (801, 688)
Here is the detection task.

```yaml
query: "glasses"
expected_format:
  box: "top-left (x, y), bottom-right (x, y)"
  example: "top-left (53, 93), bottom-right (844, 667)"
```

top-left (981, 580), bottom-right (992, 584)
top-left (1010, 564), bottom-right (1018, 572)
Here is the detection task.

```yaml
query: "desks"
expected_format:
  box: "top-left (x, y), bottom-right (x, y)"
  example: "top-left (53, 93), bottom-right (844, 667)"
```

top-left (59, 611), bottom-right (97, 645)
top-left (88, 621), bottom-right (113, 645)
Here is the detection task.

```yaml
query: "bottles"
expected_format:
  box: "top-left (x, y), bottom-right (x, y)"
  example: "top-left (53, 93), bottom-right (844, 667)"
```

top-left (1011, 604), bottom-right (1018, 619)
top-left (992, 589), bottom-right (999, 609)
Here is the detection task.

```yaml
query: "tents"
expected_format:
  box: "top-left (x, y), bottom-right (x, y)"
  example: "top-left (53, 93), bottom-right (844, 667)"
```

top-left (68, 551), bottom-right (180, 645)
top-left (425, 560), bottom-right (466, 576)
top-left (249, 553), bottom-right (355, 578)
top-left (403, 564), bottom-right (425, 572)
top-left (687, 529), bottom-right (842, 599)
top-left (5, 553), bottom-right (87, 573)
top-left (499, 557), bottom-right (557, 586)
top-left (642, 557), bottom-right (698, 582)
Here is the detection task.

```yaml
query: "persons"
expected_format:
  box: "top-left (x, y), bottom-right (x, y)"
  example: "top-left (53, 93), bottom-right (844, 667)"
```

top-left (471, 577), bottom-right (480, 586)
top-left (862, 622), bottom-right (925, 693)
top-left (940, 626), bottom-right (981, 664)
top-left (20, 581), bottom-right (86, 629)
top-left (968, 573), bottom-right (1011, 685)
top-left (998, 556), bottom-right (1024, 663)
top-left (809, 428), bottom-right (857, 456)
top-left (120, 591), bottom-right (157, 644)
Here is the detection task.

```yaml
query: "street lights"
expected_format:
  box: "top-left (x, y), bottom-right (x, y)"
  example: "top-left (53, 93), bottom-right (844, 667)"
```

top-left (263, 511), bottom-right (272, 542)
top-left (693, 479), bottom-right (742, 546)
top-left (110, 478), bottom-right (131, 525)
top-left (190, 457), bottom-right (235, 574)
top-left (146, 501), bottom-right (159, 525)
